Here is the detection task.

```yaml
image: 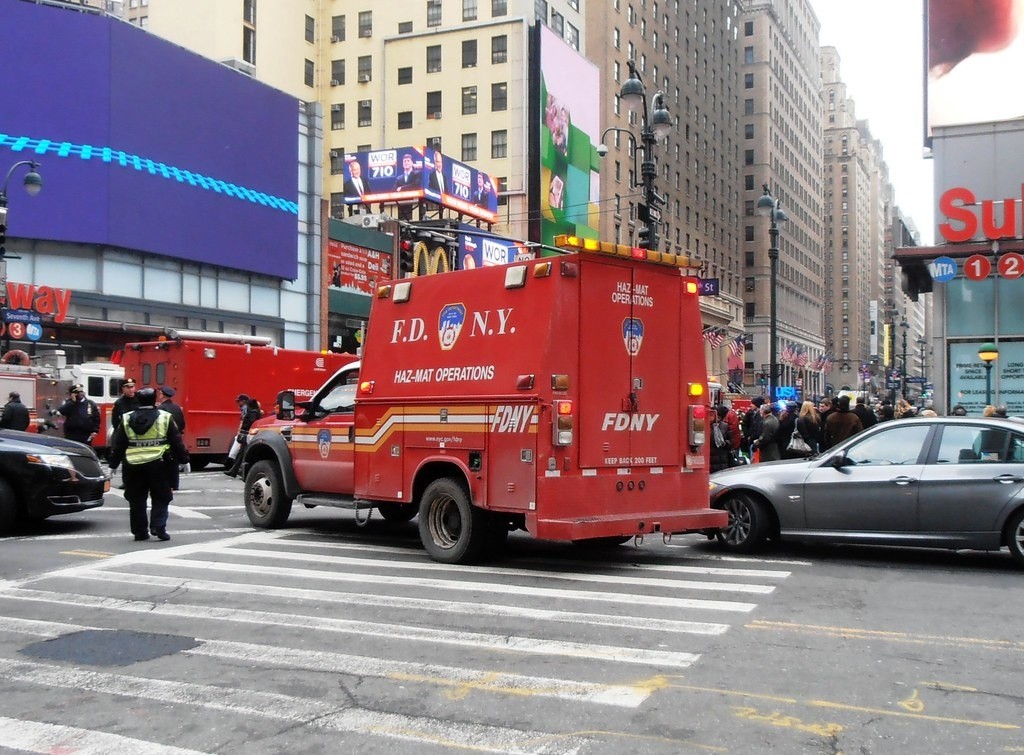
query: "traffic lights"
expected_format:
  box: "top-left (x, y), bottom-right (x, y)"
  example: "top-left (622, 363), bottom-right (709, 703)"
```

top-left (638, 229), bottom-right (649, 249)
top-left (760, 374), bottom-right (763, 382)
top-left (399, 237), bottom-right (413, 272)
top-left (922, 391), bottom-right (926, 399)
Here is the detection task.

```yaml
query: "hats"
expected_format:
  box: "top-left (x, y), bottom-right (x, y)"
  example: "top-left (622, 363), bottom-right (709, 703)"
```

top-left (236, 394), bottom-right (249, 401)
top-left (159, 386), bottom-right (175, 397)
top-left (134, 387), bottom-right (157, 405)
top-left (69, 384), bottom-right (84, 392)
top-left (751, 396), bottom-right (764, 408)
top-left (121, 378), bottom-right (136, 387)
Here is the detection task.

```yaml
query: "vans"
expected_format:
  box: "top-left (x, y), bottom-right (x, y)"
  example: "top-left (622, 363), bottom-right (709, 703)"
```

top-left (707, 377), bottom-right (726, 408)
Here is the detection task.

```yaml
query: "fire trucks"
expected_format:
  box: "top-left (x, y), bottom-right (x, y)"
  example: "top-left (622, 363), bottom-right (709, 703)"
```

top-left (0, 361), bottom-right (126, 462)
top-left (123, 327), bottom-right (362, 469)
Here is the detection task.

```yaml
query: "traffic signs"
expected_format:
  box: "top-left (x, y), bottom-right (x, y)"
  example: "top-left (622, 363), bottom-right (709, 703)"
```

top-left (646, 204), bottom-right (662, 225)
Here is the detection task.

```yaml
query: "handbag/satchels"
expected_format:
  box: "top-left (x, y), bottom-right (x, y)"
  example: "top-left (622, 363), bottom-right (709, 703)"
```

top-left (714, 425), bottom-right (724, 448)
top-left (786, 418), bottom-right (813, 453)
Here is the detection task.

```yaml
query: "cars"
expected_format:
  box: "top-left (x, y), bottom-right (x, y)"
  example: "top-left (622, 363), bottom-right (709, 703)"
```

top-left (698, 416), bottom-right (1024, 565)
top-left (0, 429), bottom-right (111, 537)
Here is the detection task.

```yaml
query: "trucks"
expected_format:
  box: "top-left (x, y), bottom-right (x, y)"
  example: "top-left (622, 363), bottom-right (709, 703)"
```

top-left (240, 237), bottom-right (730, 566)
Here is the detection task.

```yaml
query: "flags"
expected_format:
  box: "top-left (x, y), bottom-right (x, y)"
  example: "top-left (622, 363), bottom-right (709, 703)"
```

top-left (783, 345), bottom-right (808, 367)
top-left (813, 352), bottom-right (835, 374)
top-left (737, 339), bottom-right (745, 357)
top-left (728, 335), bottom-right (742, 356)
top-left (703, 327), bottom-right (716, 344)
top-left (711, 329), bottom-right (726, 351)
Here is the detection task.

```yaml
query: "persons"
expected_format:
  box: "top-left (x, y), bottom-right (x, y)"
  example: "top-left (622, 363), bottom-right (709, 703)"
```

top-left (224, 394), bottom-right (262, 479)
top-left (428, 151), bottom-right (448, 193)
top-left (343, 160), bottom-right (371, 197)
top-left (710, 394), bottom-right (1017, 474)
top-left (547, 95), bottom-right (570, 158)
top-left (394, 154), bottom-right (422, 191)
top-left (58, 384), bottom-right (101, 446)
top-left (473, 173), bottom-right (489, 209)
top-left (109, 378), bottom-right (190, 540)
top-left (0, 392), bottom-right (30, 431)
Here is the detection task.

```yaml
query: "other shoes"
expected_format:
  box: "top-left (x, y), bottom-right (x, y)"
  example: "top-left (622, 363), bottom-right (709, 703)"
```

top-left (135, 533), bottom-right (150, 541)
top-left (224, 471), bottom-right (237, 478)
top-left (150, 528), bottom-right (169, 540)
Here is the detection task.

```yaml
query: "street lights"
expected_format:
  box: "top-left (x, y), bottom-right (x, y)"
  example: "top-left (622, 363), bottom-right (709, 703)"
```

top-left (620, 60), bottom-right (674, 250)
top-left (812, 372), bottom-right (819, 404)
top-left (757, 183), bottom-right (789, 402)
top-left (1, 160), bottom-right (44, 353)
top-left (977, 343), bottom-right (999, 406)
top-left (917, 335), bottom-right (927, 408)
top-left (889, 302), bottom-right (898, 409)
top-left (860, 369), bottom-right (869, 400)
top-left (900, 315), bottom-right (910, 397)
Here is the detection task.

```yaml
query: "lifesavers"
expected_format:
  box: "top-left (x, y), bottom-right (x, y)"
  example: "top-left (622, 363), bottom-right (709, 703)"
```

top-left (0, 349), bottom-right (30, 366)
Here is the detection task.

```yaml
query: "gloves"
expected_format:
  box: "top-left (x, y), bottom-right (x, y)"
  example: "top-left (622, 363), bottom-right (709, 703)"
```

top-left (179, 462), bottom-right (190, 474)
top-left (108, 467), bottom-right (116, 479)
